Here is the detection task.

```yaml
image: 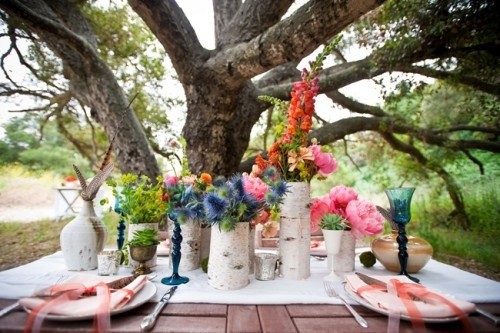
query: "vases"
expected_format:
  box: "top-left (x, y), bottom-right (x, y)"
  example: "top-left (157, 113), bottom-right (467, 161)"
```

top-left (280, 182), bottom-right (310, 280)
top-left (167, 215), bottom-right (200, 271)
top-left (60, 201), bottom-right (107, 271)
top-left (98, 251), bottom-right (121, 275)
top-left (372, 231), bottom-right (433, 274)
top-left (250, 223), bottom-right (255, 276)
top-left (336, 230), bottom-right (355, 272)
top-left (207, 222), bottom-right (249, 290)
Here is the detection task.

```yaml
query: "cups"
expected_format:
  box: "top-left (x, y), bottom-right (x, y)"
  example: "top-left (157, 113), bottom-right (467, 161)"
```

top-left (254, 253), bottom-right (279, 280)
top-left (96, 250), bottom-right (122, 275)
top-left (128, 240), bottom-right (161, 275)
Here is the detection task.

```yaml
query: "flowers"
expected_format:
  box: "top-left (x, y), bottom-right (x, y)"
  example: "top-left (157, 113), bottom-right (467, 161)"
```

top-left (309, 185), bottom-right (384, 239)
top-left (72, 163), bottom-right (114, 200)
top-left (375, 206), bottom-right (399, 230)
top-left (205, 166), bottom-right (288, 231)
top-left (252, 34), bottom-right (337, 182)
top-left (163, 174), bottom-right (212, 222)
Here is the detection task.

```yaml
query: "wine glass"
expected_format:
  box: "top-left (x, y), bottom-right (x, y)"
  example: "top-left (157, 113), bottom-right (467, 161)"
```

top-left (161, 180), bottom-right (198, 285)
top-left (112, 182), bottom-right (130, 262)
top-left (384, 186), bottom-right (421, 283)
top-left (317, 229), bottom-right (348, 285)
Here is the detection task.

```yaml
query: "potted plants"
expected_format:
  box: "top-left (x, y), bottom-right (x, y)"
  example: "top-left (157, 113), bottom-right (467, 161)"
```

top-left (319, 213), bottom-right (350, 282)
top-left (106, 174), bottom-right (167, 242)
top-left (127, 228), bottom-right (161, 276)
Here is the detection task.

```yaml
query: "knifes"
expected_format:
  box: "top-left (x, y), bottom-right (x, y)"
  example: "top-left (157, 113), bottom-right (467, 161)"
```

top-left (354, 270), bottom-right (424, 302)
top-left (140, 286), bottom-right (180, 331)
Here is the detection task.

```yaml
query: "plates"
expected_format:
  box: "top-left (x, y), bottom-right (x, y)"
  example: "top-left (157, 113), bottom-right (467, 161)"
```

top-left (343, 275), bottom-right (470, 322)
top-left (21, 273), bottom-right (157, 321)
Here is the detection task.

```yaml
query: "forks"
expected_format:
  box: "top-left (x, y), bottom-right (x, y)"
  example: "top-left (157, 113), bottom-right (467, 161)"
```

top-left (320, 279), bottom-right (368, 329)
